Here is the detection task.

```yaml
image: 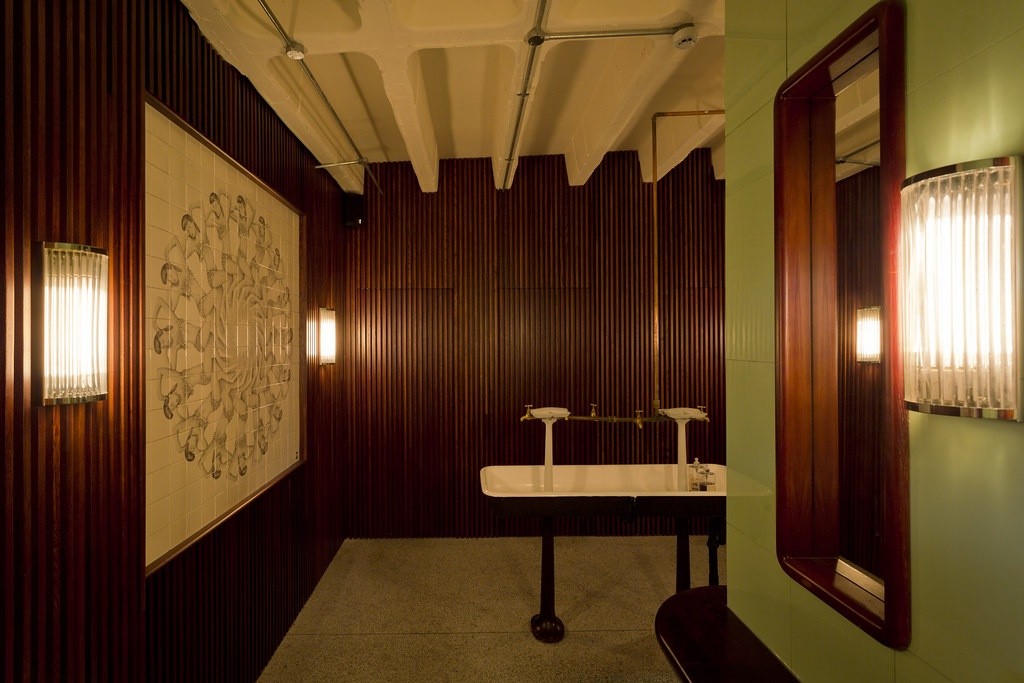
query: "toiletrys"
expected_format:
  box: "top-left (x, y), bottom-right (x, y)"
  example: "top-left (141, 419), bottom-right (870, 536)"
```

top-left (688, 457), bottom-right (716, 492)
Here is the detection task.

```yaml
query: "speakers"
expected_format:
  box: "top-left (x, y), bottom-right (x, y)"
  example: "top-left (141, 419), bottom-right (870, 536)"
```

top-left (341, 192), bottom-right (366, 226)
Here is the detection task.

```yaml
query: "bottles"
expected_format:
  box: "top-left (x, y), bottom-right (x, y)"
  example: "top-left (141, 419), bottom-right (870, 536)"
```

top-left (689, 457), bottom-right (716, 492)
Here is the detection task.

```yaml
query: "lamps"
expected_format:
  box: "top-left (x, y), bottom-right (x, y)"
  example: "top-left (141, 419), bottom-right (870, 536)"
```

top-left (42, 240), bottom-right (109, 405)
top-left (318, 308), bottom-right (336, 365)
top-left (855, 305), bottom-right (881, 363)
top-left (895, 151), bottom-right (1024, 420)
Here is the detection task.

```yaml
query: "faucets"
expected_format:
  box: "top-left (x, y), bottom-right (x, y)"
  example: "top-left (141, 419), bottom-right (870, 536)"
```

top-left (590, 403), bottom-right (597, 416)
top-left (697, 406), bottom-right (710, 422)
top-left (636, 410), bottom-right (643, 429)
top-left (520, 404), bottom-right (535, 422)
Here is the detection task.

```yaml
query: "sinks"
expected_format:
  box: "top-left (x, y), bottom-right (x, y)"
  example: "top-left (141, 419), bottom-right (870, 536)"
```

top-left (477, 464), bottom-right (772, 503)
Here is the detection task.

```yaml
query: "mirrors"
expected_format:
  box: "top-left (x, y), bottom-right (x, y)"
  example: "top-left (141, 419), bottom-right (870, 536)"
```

top-left (773, 0), bottom-right (910, 651)
